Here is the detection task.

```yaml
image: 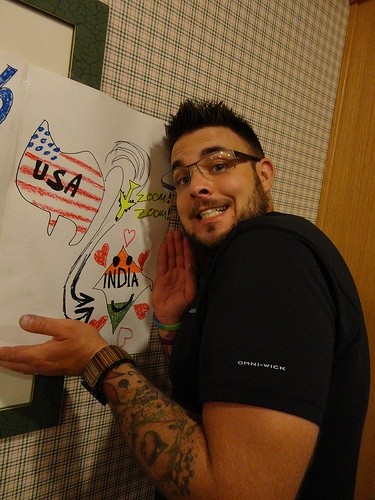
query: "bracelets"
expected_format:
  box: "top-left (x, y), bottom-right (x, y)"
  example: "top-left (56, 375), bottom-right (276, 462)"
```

top-left (152, 315), bottom-right (183, 331)
top-left (80, 345), bottom-right (138, 406)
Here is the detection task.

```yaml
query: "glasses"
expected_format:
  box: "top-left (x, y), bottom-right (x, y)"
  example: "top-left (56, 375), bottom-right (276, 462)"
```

top-left (161, 148), bottom-right (262, 194)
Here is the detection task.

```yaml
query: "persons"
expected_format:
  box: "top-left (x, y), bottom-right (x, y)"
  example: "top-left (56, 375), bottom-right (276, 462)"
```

top-left (0, 98), bottom-right (370, 500)
